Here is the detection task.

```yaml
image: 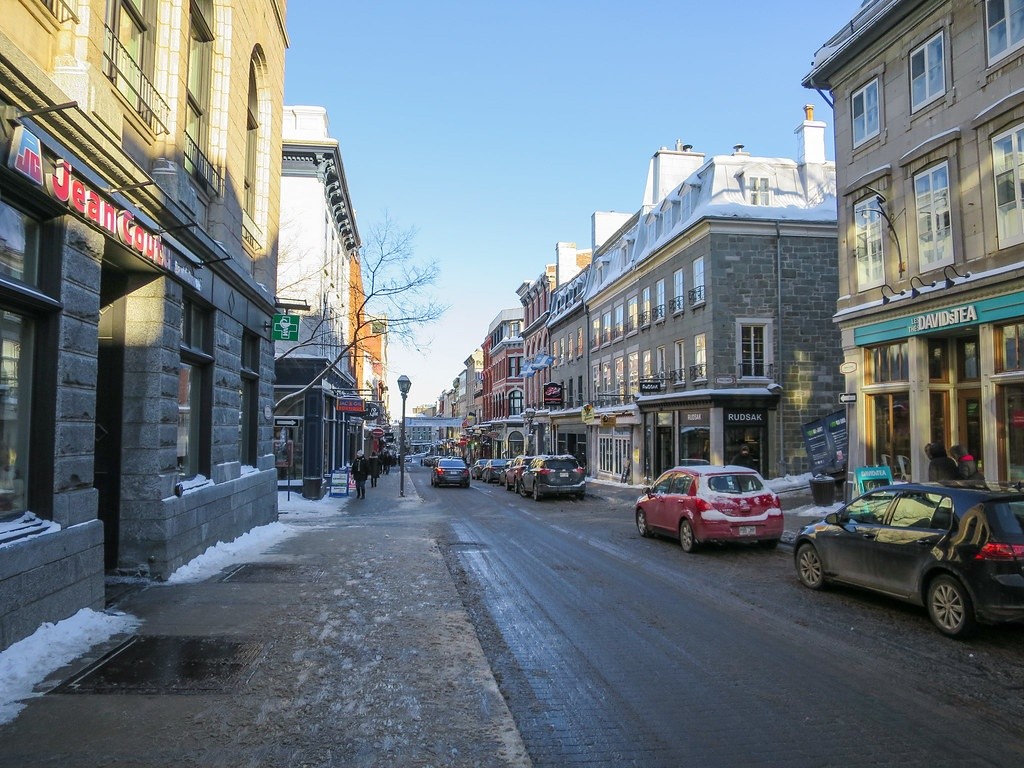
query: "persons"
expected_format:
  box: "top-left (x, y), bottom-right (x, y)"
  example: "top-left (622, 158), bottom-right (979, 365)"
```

top-left (926, 442), bottom-right (957, 482)
top-left (730, 444), bottom-right (756, 490)
top-left (374, 445), bottom-right (397, 475)
top-left (462, 451), bottom-right (470, 465)
top-left (369, 452), bottom-right (382, 487)
top-left (351, 450), bottom-right (371, 499)
top-left (950, 445), bottom-right (985, 480)
top-left (525, 451), bottom-right (529, 456)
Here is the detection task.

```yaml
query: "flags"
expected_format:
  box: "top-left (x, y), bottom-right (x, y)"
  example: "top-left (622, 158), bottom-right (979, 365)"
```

top-left (517, 354), bottom-right (555, 378)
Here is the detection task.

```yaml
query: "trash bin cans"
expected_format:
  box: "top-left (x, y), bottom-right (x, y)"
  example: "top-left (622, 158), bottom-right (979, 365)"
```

top-left (809, 474), bottom-right (836, 507)
top-left (303, 475), bottom-right (322, 499)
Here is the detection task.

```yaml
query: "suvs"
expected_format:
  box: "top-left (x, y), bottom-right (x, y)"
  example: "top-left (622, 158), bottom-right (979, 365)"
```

top-left (518, 455), bottom-right (586, 501)
top-left (505, 456), bottom-right (536, 492)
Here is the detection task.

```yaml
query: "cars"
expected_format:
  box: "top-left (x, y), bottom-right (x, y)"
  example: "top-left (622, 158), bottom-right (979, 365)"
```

top-left (430, 458), bottom-right (470, 488)
top-left (424, 455), bottom-right (444, 468)
top-left (498, 460), bottom-right (515, 485)
top-left (633, 465), bottom-right (783, 553)
top-left (471, 459), bottom-right (490, 480)
top-left (481, 459), bottom-right (508, 484)
top-left (404, 456), bottom-right (413, 462)
top-left (792, 479), bottom-right (1024, 640)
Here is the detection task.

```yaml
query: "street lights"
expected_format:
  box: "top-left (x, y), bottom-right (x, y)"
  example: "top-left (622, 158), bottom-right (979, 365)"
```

top-left (397, 375), bottom-right (412, 497)
top-left (526, 408), bottom-right (536, 455)
top-left (469, 429), bottom-right (473, 460)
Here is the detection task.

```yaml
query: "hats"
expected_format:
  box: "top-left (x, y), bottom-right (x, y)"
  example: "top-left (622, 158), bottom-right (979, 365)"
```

top-left (372, 451), bottom-right (377, 456)
top-left (357, 449), bottom-right (362, 458)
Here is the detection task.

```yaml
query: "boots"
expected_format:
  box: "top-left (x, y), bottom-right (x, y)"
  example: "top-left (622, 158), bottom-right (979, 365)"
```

top-left (356, 489), bottom-right (361, 498)
top-left (360, 489), bottom-right (365, 499)
